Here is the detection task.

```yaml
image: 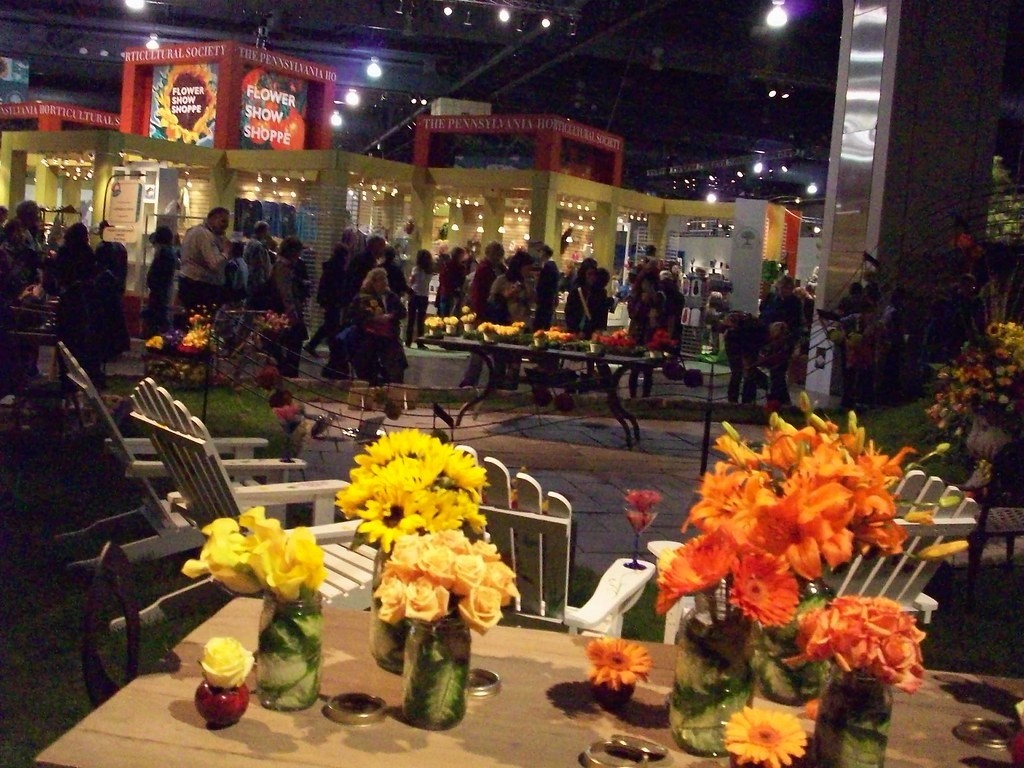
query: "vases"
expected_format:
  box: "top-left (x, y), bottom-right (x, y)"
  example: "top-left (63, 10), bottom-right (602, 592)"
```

top-left (535, 339), bottom-right (545, 349)
top-left (753, 576), bottom-right (835, 706)
top-left (255, 590), bottom-right (325, 711)
top-left (593, 680), bottom-right (635, 712)
top-left (484, 333), bottom-right (495, 343)
top-left (967, 410), bottom-right (1021, 463)
top-left (669, 593), bottom-right (760, 759)
top-left (445, 325), bottom-right (457, 335)
top-left (194, 678), bottom-right (251, 727)
top-left (400, 614), bottom-right (471, 732)
top-left (809, 664), bottom-right (893, 768)
top-left (649, 350), bottom-right (663, 358)
top-left (464, 324), bottom-right (474, 333)
top-left (370, 547), bottom-right (412, 674)
top-left (590, 344), bottom-right (602, 354)
top-left (429, 328), bottom-right (442, 336)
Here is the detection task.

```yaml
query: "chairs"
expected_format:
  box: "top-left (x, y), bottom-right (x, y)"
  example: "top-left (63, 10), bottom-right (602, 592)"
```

top-left (802, 469), bottom-right (980, 626)
top-left (54, 340), bottom-right (377, 630)
top-left (456, 444), bottom-right (656, 638)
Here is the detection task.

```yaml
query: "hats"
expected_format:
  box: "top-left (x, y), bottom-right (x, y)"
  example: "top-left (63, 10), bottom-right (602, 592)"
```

top-left (659, 270), bottom-right (671, 281)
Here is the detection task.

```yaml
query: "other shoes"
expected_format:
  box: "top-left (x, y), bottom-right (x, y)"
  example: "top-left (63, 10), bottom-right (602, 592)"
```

top-left (728, 394), bottom-right (738, 404)
top-left (304, 344), bottom-right (322, 359)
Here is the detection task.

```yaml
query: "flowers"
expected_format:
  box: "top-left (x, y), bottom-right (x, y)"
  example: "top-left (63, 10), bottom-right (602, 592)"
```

top-left (139, 304), bottom-right (291, 356)
top-left (180, 391), bottom-right (970, 768)
top-left (424, 304), bottom-right (680, 361)
top-left (923, 319), bottom-right (1024, 437)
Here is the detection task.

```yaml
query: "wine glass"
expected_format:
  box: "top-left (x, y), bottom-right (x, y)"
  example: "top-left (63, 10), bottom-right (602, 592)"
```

top-left (622, 489), bottom-right (665, 570)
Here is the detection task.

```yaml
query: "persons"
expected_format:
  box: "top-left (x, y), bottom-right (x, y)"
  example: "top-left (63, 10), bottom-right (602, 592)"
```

top-left (0, 207), bottom-right (885, 416)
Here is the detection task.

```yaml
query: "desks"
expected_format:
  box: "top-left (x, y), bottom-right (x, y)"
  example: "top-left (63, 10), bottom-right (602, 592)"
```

top-left (418, 336), bottom-right (675, 449)
top-left (35, 595), bottom-right (1023, 768)
